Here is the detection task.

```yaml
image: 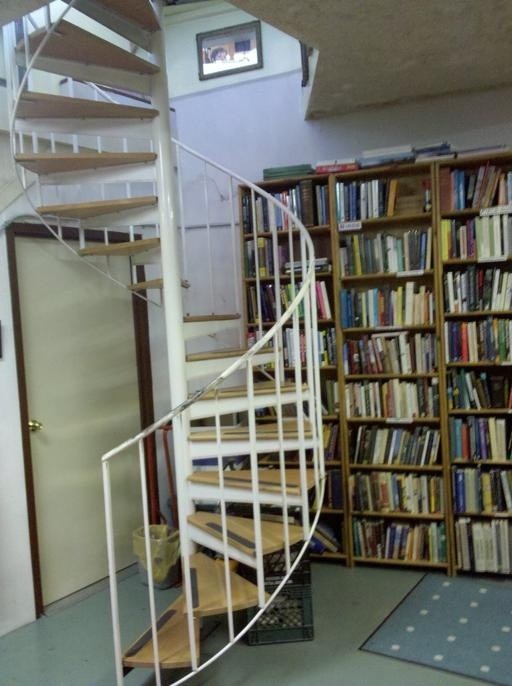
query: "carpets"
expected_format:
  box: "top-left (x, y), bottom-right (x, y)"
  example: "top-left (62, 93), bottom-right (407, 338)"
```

top-left (357, 568), bottom-right (512, 684)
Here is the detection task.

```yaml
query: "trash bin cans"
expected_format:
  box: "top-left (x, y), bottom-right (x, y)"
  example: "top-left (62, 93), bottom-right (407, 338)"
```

top-left (131, 525), bottom-right (181, 590)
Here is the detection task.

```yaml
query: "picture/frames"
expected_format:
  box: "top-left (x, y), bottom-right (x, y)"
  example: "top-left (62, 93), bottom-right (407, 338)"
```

top-left (195, 19), bottom-right (264, 81)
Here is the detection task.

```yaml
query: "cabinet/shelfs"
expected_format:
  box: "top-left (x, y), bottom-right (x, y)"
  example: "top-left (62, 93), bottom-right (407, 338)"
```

top-left (433, 151), bottom-right (512, 579)
top-left (330, 162), bottom-right (453, 579)
top-left (235, 173), bottom-right (348, 570)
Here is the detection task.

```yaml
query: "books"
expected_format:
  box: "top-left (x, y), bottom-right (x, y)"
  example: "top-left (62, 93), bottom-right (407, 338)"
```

top-left (242, 144), bottom-right (512, 576)
